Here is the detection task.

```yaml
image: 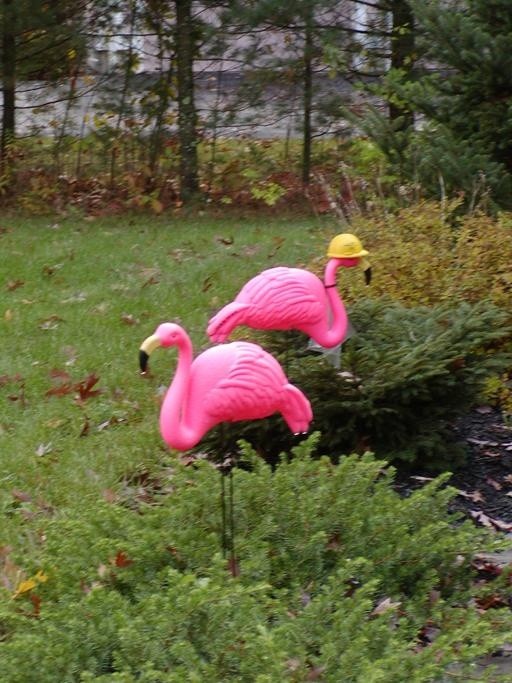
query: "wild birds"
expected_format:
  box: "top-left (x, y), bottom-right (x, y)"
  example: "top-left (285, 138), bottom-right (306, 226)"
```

top-left (206, 232), bottom-right (372, 379)
top-left (140, 322), bottom-right (315, 579)
